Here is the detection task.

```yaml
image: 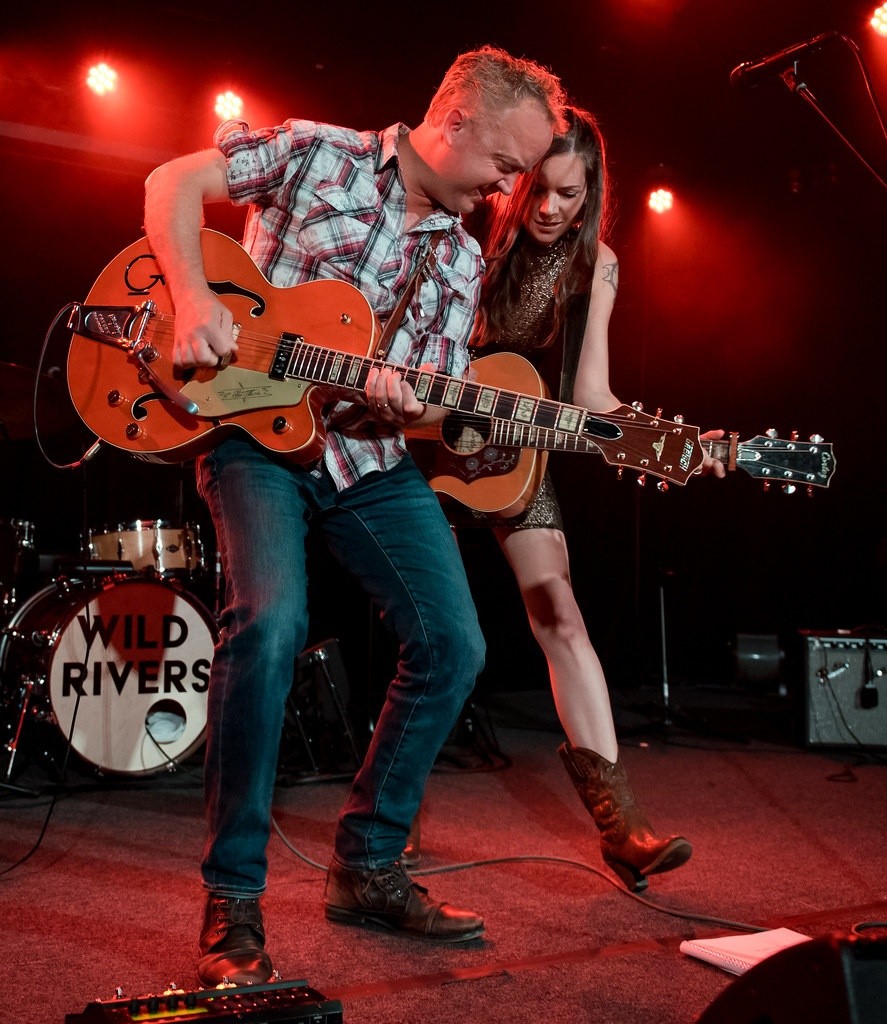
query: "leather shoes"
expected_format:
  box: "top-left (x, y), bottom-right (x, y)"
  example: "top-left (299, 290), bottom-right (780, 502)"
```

top-left (195, 890), bottom-right (273, 988)
top-left (323, 859), bottom-right (485, 944)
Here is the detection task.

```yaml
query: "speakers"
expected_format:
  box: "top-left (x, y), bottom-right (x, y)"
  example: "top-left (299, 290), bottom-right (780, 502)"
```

top-left (799, 628), bottom-right (887, 749)
top-left (696, 930), bottom-right (887, 1024)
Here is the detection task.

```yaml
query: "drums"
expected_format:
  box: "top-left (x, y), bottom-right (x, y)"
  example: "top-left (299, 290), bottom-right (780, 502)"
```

top-left (0, 517), bottom-right (36, 615)
top-left (0, 573), bottom-right (219, 777)
top-left (78, 520), bottom-right (205, 582)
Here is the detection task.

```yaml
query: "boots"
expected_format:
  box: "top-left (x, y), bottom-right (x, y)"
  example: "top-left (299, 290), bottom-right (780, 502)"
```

top-left (398, 809), bottom-right (421, 864)
top-left (559, 742), bottom-right (691, 891)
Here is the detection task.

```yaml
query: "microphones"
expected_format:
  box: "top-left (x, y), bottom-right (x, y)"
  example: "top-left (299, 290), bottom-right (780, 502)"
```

top-left (860, 653), bottom-right (878, 709)
top-left (730, 31), bottom-right (837, 93)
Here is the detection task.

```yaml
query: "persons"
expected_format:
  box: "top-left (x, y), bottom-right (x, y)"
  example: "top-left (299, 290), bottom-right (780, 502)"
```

top-left (403, 104), bottom-right (724, 893)
top-left (144, 44), bottom-right (569, 991)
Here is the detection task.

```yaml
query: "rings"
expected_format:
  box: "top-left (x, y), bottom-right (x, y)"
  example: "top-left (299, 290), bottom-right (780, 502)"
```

top-left (377, 403), bottom-right (389, 408)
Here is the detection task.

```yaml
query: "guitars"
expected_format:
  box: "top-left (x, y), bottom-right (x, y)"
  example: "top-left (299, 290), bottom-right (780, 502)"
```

top-left (402, 351), bottom-right (836, 520)
top-left (66, 226), bottom-right (705, 492)
